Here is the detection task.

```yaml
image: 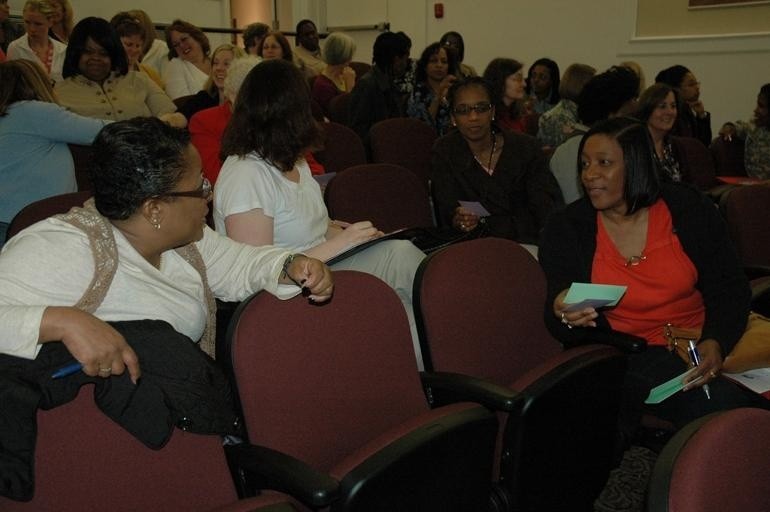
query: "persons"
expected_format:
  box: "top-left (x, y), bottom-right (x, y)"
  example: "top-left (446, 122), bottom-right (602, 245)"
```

top-left (440, 31), bottom-right (477, 80)
top-left (1, 59), bottom-right (116, 248)
top-left (0, 117), bottom-right (334, 385)
top-left (406, 43), bottom-right (457, 136)
top-left (53, 17), bottom-right (188, 128)
top-left (0, 0), bottom-right (73, 81)
top-left (350, 32), bottom-right (416, 145)
top-left (482, 59), bottom-right (711, 183)
top-left (108, 7), bottom-right (357, 192)
top-left (719, 84), bottom-right (770, 179)
top-left (214, 60), bottom-right (428, 372)
top-left (538, 117), bottom-right (770, 418)
top-left (430, 78), bottom-right (564, 244)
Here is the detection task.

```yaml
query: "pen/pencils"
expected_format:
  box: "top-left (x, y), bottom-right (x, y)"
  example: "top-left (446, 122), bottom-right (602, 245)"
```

top-left (47, 362), bottom-right (81, 380)
top-left (687, 339), bottom-right (711, 400)
top-left (331, 219), bottom-right (384, 238)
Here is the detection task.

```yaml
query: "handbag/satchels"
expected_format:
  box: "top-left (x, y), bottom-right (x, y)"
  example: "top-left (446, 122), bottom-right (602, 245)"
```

top-left (415, 224), bottom-right (494, 255)
top-left (663, 312), bottom-right (770, 373)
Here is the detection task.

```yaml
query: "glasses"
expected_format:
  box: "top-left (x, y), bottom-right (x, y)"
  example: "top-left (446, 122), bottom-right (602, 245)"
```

top-left (451, 100), bottom-right (492, 116)
top-left (150, 175), bottom-right (213, 205)
top-left (682, 80), bottom-right (700, 88)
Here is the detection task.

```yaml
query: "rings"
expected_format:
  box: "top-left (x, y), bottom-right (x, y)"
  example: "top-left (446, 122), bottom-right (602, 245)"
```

top-left (709, 369), bottom-right (716, 379)
top-left (460, 221), bottom-right (465, 229)
top-left (98, 368), bottom-right (112, 373)
top-left (560, 312), bottom-right (572, 329)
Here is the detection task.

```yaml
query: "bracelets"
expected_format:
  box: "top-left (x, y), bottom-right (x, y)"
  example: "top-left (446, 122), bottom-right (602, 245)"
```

top-left (283, 253), bottom-right (307, 272)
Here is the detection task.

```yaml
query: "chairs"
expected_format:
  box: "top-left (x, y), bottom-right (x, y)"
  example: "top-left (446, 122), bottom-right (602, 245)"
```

top-left (171, 94), bottom-right (196, 108)
top-left (646, 407), bottom-right (769, 512)
top-left (312, 122), bottom-right (366, 173)
top-left (413, 237), bottom-right (648, 512)
top-left (351, 61), bottom-right (370, 77)
top-left (225, 270), bottom-right (524, 512)
top-left (0, 384), bottom-right (343, 511)
top-left (672, 136), bottom-right (746, 189)
top-left (5, 191), bottom-right (91, 243)
top-left (720, 184), bottom-right (770, 300)
top-left (324, 164), bottom-right (436, 234)
top-left (366, 116), bottom-right (444, 176)
